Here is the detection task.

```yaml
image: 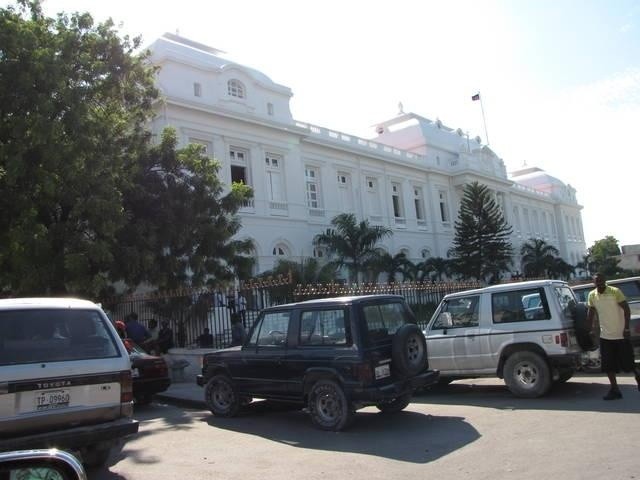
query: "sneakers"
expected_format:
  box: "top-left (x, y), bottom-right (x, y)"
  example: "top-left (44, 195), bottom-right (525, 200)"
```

top-left (603, 388), bottom-right (622, 400)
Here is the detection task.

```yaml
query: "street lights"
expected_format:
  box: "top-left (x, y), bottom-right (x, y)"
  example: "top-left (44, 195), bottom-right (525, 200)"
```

top-left (471, 92), bottom-right (490, 146)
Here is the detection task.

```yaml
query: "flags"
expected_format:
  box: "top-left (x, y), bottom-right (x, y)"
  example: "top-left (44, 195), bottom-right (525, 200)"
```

top-left (472, 94), bottom-right (479, 101)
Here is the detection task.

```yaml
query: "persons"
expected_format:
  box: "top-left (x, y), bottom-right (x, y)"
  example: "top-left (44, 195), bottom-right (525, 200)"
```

top-left (126, 312), bottom-right (152, 345)
top-left (230, 313), bottom-right (247, 347)
top-left (144, 320), bottom-right (159, 356)
top-left (585, 273), bottom-right (640, 400)
top-left (196, 328), bottom-right (213, 348)
top-left (154, 320), bottom-right (172, 355)
top-left (114, 320), bottom-right (128, 339)
top-left (32, 314), bottom-right (65, 339)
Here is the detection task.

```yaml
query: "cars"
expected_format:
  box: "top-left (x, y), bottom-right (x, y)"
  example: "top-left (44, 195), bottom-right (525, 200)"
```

top-left (0, 297), bottom-right (170, 480)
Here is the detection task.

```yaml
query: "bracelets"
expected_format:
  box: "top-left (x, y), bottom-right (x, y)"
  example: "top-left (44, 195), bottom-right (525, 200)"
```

top-left (624, 328), bottom-right (630, 333)
